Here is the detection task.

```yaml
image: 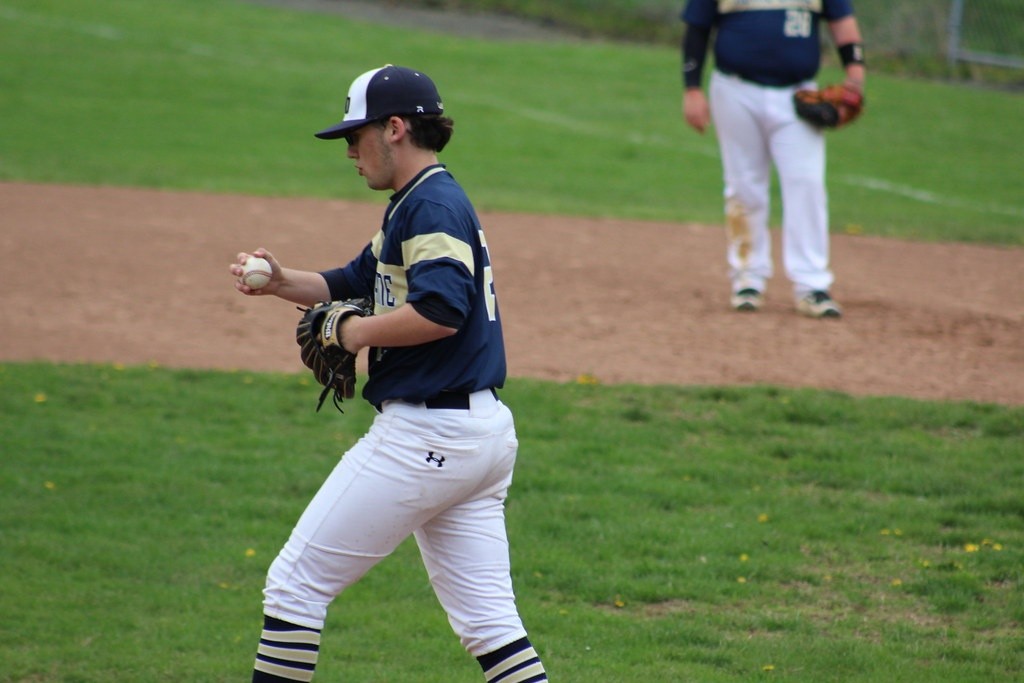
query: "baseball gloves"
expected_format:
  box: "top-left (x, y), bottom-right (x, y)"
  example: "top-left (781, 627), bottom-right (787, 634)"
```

top-left (794, 86), bottom-right (864, 127)
top-left (295, 296), bottom-right (368, 401)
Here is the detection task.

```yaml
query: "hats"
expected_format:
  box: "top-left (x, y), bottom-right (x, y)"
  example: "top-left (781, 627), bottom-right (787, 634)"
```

top-left (314, 64), bottom-right (444, 140)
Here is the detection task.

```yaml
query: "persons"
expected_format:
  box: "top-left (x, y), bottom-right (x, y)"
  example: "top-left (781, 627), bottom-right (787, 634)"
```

top-left (680, 0), bottom-right (867, 321)
top-left (226, 61), bottom-right (555, 683)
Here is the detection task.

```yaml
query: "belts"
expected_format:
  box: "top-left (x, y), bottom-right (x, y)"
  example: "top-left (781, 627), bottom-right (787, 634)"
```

top-left (374, 386), bottom-right (498, 414)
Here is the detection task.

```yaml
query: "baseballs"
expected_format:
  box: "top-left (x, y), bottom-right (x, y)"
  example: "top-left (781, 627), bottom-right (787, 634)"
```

top-left (237, 257), bottom-right (272, 291)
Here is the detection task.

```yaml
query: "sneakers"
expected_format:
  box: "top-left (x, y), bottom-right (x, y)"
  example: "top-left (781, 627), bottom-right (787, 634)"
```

top-left (798, 290), bottom-right (840, 317)
top-left (733, 288), bottom-right (765, 312)
